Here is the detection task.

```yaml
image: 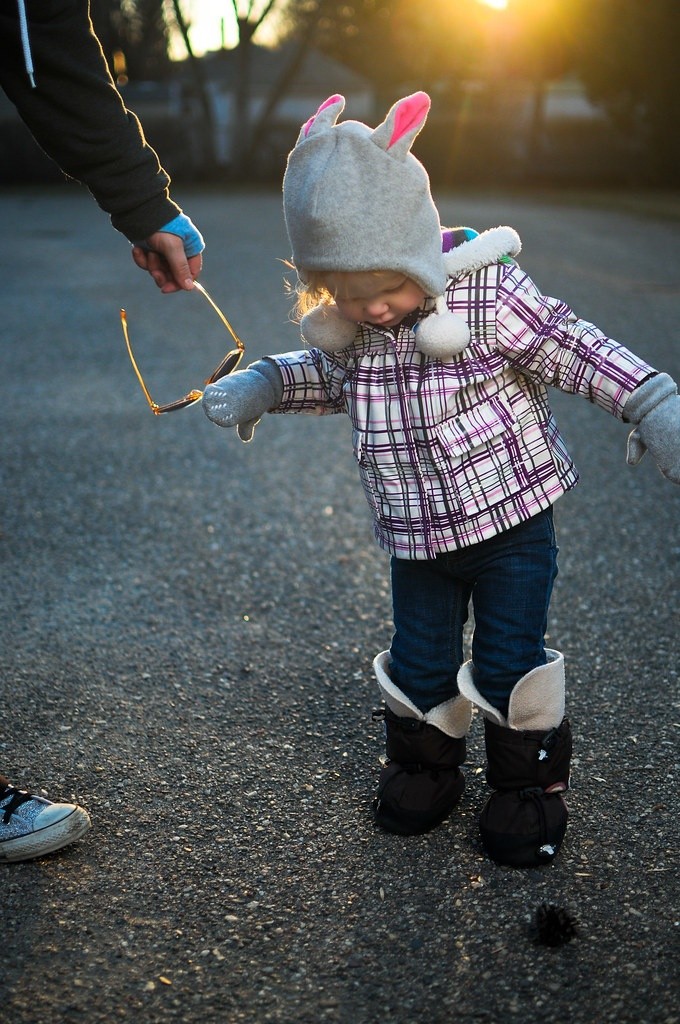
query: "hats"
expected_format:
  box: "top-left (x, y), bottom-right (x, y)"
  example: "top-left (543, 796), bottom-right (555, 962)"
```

top-left (282, 89), bottom-right (471, 358)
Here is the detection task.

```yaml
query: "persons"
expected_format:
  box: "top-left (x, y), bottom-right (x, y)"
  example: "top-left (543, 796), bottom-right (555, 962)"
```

top-left (0, 0), bottom-right (204, 862)
top-left (204, 91), bottom-right (679, 866)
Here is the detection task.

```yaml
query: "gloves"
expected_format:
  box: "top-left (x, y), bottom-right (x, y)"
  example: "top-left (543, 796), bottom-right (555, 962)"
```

top-left (202, 356), bottom-right (284, 443)
top-left (622, 371), bottom-right (680, 484)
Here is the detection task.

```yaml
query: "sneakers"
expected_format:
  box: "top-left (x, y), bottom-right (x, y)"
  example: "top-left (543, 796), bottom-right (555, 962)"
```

top-left (0, 779), bottom-right (91, 866)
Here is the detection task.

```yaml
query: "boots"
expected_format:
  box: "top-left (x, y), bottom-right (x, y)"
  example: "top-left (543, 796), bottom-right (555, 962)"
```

top-left (372, 649), bottom-right (475, 829)
top-left (457, 648), bottom-right (573, 858)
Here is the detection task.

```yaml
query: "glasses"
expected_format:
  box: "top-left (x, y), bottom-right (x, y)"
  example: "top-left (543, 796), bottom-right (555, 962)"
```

top-left (120, 279), bottom-right (245, 415)
